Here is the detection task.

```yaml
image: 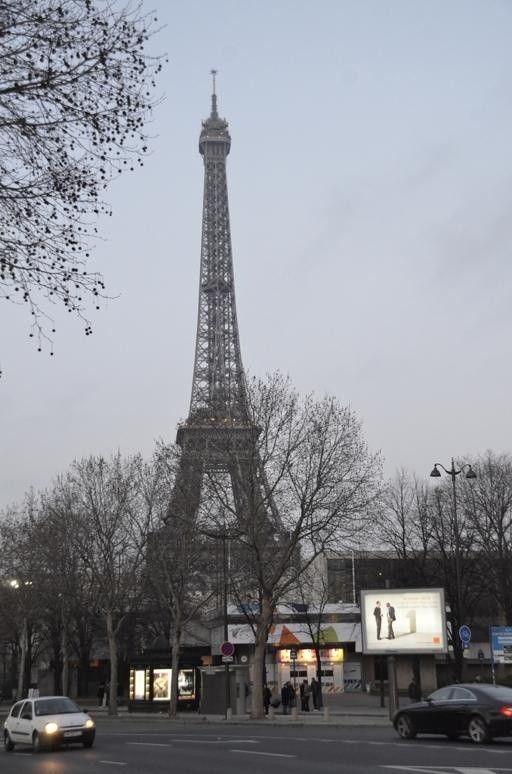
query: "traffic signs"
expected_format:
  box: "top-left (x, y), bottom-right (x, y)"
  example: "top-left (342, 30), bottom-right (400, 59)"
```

top-left (491, 626), bottom-right (512, 650)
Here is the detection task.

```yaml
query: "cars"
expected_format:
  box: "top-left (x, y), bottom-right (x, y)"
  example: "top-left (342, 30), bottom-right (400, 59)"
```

top-left (392, 683), bottom-right (512, 743)
top-left (3, 696), bottom-right (96, 753)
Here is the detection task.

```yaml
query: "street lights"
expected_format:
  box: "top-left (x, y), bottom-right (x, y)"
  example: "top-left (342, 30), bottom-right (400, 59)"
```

top-left (429, 457), bottom-right (479, 683)
top-left (8, 576), bottom-right (35, 700)
top-left (348, 549), bottom-right (356, 603)
top-left (158, 512), bottom-right (279, 720)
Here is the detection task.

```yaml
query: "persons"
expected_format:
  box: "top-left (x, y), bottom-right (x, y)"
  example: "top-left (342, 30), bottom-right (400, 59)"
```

top-left (408, 679), bottom-right (417, 703)
top-left (384, 603), bottom-right (395, 639)
top-left (264, 678), bottom-right (318, 715)
top-left (373, 601), bottom-right (383, 641)
top-left (97, 681), bottom-right (121, 706)
